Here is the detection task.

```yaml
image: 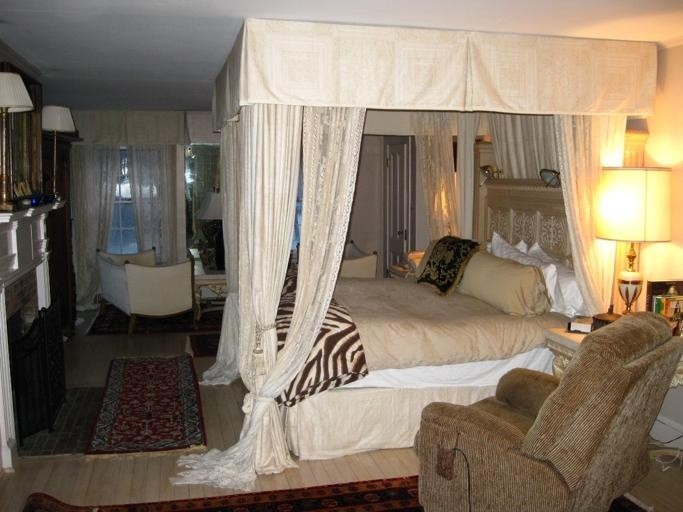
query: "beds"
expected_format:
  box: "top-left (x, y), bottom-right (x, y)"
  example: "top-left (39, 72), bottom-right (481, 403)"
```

top-left (168, 17), bottom-right (658, 491)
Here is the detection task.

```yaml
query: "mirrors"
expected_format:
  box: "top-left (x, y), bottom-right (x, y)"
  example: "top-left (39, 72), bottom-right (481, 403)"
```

top-left (0, 61), bottom-right (43, 203)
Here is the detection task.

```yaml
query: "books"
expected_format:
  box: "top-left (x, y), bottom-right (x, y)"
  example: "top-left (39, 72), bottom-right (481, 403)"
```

top-left (565, 313), bottom-right (592, 335)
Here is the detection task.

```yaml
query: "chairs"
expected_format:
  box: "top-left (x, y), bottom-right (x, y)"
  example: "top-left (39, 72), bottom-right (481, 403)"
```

top-left (296, 240), bottom-right (377, 280)
top-left (415, 310), bottom-right (683, 511)
top-left (94, 247), bottom-right (194, 337)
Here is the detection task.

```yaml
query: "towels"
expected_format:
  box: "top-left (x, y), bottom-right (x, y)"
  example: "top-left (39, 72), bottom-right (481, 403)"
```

top-left (276, 272), bottom-right (367, 405)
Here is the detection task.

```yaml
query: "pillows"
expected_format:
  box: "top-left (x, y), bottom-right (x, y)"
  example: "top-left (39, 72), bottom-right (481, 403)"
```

top-left (412, 229), bottom-right (586, 320)
top-left (523, 312), bottom-right (671, 459)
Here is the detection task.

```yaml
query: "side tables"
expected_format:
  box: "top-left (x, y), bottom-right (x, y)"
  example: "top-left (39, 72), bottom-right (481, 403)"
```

top-left (192, 272), bottom-right (228, 320)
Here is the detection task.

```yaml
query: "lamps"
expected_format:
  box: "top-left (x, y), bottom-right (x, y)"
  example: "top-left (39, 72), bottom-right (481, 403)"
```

top-left (0, 72), bottom-right (34, 210)
top-left (194, 183), bottom-right (225, 270)
top-left (479, 165), bottom-right (502, 180)
top-left (540, 168), bottom-right (561, 188)
top-left (42, 104), bottom-right (77, 203)
top-left (594, 166), bottom-right (671, 314)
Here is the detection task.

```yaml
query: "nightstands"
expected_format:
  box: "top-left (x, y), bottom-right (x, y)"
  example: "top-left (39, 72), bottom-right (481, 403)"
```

top-left (548, 327), bottom-right (683, 387)
top-left (387, 263), bottom-right (414, 279)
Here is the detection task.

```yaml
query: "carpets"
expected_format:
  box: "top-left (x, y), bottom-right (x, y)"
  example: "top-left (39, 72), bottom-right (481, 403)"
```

top-left (83, 353), bottom-right (208, 458)
top-left (86, 302), bottom-right (229, 335)
top-left (21, 474), bottom-right (658, 512)
